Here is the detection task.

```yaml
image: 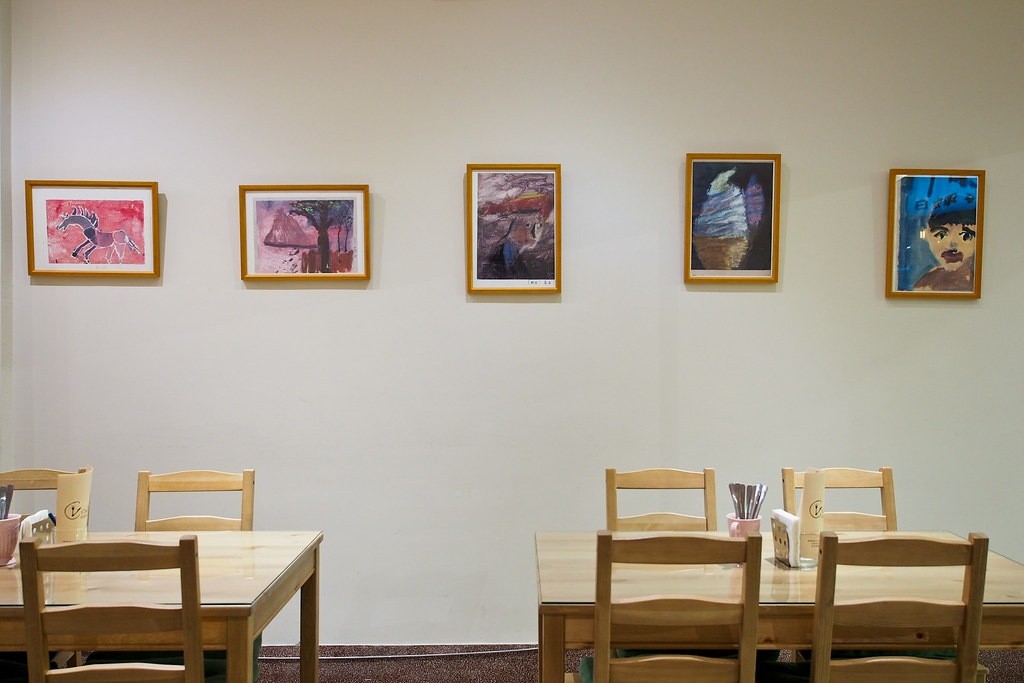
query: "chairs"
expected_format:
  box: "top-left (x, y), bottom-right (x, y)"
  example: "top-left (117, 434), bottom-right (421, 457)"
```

top-left (0, 467), bottom-right (92, 530)
top-left (592, 528), bottom-right (764, 683)
top-left (604, 466), bottom-right (719, 531)
top-left (18, 534), bottom-right (208, 683)
top-left (780, 465), bottom-right (899, 531)
top-left (135, 467), bottom-right (256, 531)
top-left (808, 530), bottom-right (990, 683)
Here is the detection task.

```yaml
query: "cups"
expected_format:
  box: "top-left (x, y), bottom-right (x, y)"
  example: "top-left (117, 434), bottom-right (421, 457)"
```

top-left (726, 513), bottom-right (762, 567)
top-left (0, 514), bottom-right (22, 565)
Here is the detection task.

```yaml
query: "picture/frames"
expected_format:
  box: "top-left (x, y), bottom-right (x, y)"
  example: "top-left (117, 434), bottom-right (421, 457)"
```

top-left (885, 168), bottom-right (986, 301)
top-left (24, 180), bottom-right (161, 279)
top-left (466, 163), bottom-right (562, 295)
top-left (238, 184), bottom-right (371, 282)
top-left (682, 152), bottom-right (783, 285)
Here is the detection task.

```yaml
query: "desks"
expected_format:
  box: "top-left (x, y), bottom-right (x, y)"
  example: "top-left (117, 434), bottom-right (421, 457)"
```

top-left (534, 529), bottom-right (1024, 683)
top-left (0, 531), bottom-right (322, 683)
